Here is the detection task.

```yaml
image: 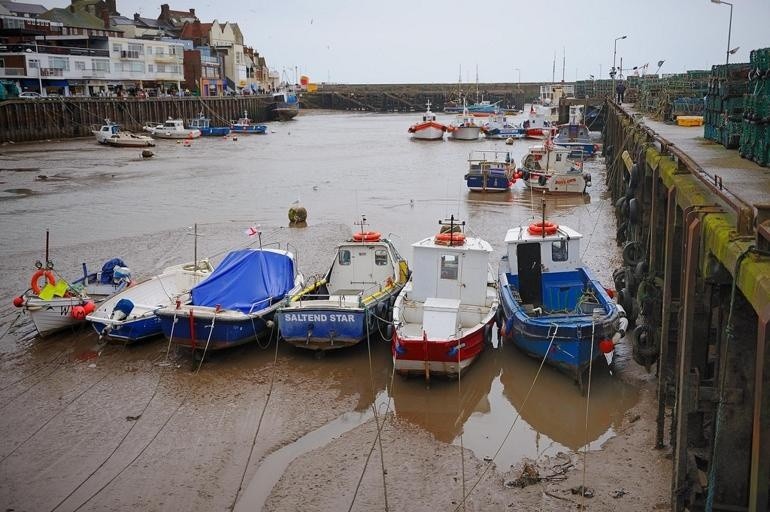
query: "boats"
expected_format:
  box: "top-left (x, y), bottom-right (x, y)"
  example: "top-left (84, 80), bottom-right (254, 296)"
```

top-left (92, 119), bottom-right (155, 145)
top-left (86, 224), bottom-right (212, 342)
top-left (185, 106), bottom-right (231, 135)
top-left (523, 102), bottom-right (557, 138)
top-left (444, 94), bottom-right (519, 117)
top-left (483, 104), bottom-right (524, 138)
top-left (552, 104), bottom-right (596, 162)
top-left (154, 225), bottom-right (306, 351)
top-left (230, 109), bottom-right (267, 132)
top-left (464, 152), bottom-right (519, 193)
top-left (14, 229), bottom-right (133, 338)
top-left (519, 133), bottom-right (592, 194)
top-left (408, 99), bottom-right (448, 139)
top-left (505, 198), bottom-right (629, 394)
top-left (277, 217), bottom-right (410, 352)
top-left (391, 216), bottom-right (501, 378)
top-left (448, 97), bottom-right (483, 140)
top-left (143, 116), bottom-right (202, 138)
top-left (266, 94), bottom-right (299, 122)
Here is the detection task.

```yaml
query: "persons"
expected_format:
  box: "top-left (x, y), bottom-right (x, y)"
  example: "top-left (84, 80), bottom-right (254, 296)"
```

top-left (616, 80), bottom-right (627, 102)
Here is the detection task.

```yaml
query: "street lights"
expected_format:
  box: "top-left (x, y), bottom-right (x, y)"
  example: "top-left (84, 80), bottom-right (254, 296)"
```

top-left (612, 35), bottom-right (628, 97)
top-left (709, 0), bottom-right (733, 63)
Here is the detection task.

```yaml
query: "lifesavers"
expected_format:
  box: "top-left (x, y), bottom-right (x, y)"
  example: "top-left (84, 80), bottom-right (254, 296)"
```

top-left (31, 272), bottom-right (55, 295)
top-left (435, 232), bottom-right (466, 242)
top-left (527, 227), bottom-right (556, 235)
top-left (353, 232), bottom-right (381, 240)
top-left (530, 221), bottom-right (556, 231)
